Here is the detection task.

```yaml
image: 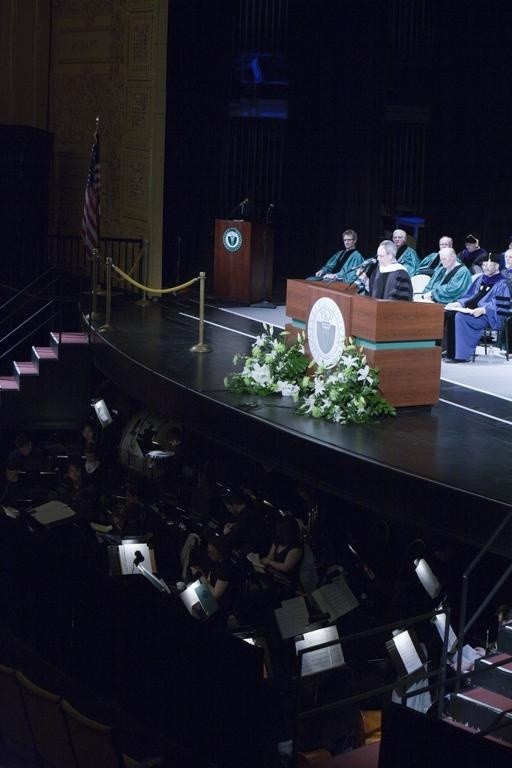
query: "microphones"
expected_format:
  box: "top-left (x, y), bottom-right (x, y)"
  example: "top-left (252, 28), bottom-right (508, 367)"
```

top-left (345, 259), bottom-right (377, 291)
top-left (239, 198), bottom-right (248, 206)
top-left (267, 203), bottom-right (274, 213)
top-left (325, 261), bottom-right (369, 288)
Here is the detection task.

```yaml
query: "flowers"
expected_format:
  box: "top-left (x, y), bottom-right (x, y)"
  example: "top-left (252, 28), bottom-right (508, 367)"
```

top-left (223, 320), bottom-right (310, 399)
top-left (293, 336), bottom-right (398, 426)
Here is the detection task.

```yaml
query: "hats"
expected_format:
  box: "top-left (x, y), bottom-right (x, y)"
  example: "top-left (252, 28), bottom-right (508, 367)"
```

top-left (482, 252), bottom-right (500, 264)
top-left (464, 233), bottom-right (480, 247)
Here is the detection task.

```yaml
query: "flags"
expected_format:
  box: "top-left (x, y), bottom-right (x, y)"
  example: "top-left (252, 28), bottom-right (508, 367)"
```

top-left (80, 129), bottom-right (101, 257)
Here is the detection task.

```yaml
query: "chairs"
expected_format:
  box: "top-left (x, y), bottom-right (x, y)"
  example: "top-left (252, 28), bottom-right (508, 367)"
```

top-left (469, 292), bottom-right (512, 363)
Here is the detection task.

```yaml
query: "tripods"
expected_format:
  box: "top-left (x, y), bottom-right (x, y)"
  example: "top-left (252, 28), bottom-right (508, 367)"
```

top-left (250, 219), bottom-right (277, 309)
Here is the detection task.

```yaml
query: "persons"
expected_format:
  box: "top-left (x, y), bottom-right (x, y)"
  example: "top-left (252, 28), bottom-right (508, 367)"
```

top-left (356, 240), bottom-right (412, 301)
top-left (419, 236), bottom-right (462, 277)
top-left (305, 230), bottom-right (364, 285)
top-left (422, 248), bottom-right (472, 306)
top-left (500, 249), bottom-right (512, 282)
top-left (392, 229), bottom-right (419, 277)
top-left (442, 253), bottom-right (512, 363)
top-left (457, 232), bottom-right (488, 275)
